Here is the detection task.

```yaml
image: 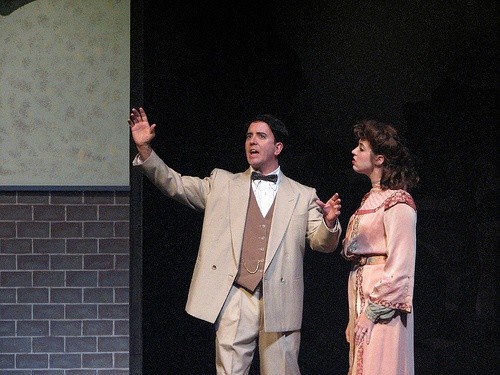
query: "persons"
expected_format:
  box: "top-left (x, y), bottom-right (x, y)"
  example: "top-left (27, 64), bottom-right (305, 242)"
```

top-left (341, 119), bottom-right (416, 375)
top-left (128, 107), bottom-right (342, 375)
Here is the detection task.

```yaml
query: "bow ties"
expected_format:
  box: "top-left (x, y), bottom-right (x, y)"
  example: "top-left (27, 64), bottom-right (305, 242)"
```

top-left (251, 172), bottom-right (278, 182)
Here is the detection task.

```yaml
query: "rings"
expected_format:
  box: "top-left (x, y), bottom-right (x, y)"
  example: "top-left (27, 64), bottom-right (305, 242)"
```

top-left (362, 330), bottom-right (365, 334)
